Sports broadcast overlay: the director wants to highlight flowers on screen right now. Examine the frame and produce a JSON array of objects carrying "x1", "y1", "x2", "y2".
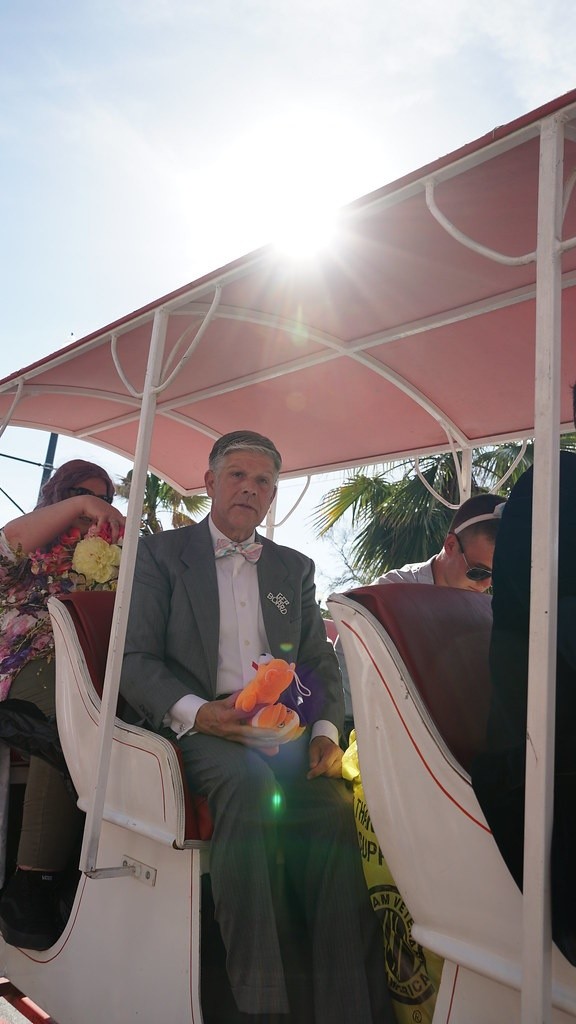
[{"x1": 3, "y1": 519, "x2": 129, "y2": 668}]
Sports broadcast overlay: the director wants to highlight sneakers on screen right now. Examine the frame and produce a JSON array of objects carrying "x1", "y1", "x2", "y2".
[{"x1": 0, "y1": 866, "x2": 64, "y2": 949}]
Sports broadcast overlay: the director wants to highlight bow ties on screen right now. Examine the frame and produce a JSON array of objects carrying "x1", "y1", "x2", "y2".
[{"x1": 214, "y1": 537, "x2": 265, "y2": 565}]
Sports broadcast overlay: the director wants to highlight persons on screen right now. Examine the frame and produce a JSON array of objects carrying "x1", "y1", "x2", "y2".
[
  {"x1": 118, "y1": 428, "x2": 397, "y2": 1024},
  {"x1": 1, "y1": 461, "x2": 131, "y2": 933},
  {"x1": 473, "y1": 381, "x2": 576, "y2": 963},
  {"x1": 332, "y1": 492, "x2": 506, "y2": 751}
]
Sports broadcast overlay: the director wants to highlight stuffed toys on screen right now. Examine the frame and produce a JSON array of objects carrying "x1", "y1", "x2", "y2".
[
  {"x1": 249, "y1": 703, "x2": 306, "y2": 745},
  {"x1": 235, "y1": 652, "x2": 296, "y2": 711}
]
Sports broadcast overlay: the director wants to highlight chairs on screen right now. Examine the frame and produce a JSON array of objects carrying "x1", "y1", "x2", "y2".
[
  {"x1": 47, "y1": 588, "x2": 224, "y2": 849},
  {"x1": 328, "y1": 575, "x2": 572, "y2": 1009}
]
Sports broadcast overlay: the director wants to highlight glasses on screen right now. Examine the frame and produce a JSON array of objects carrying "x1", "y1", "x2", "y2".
[
  {"x1": 447, "y1": 530, "x2": 495, "y2": 583},
  {"x1": 67, "y1": 486, "x2": 112, "y2": 506}
]
